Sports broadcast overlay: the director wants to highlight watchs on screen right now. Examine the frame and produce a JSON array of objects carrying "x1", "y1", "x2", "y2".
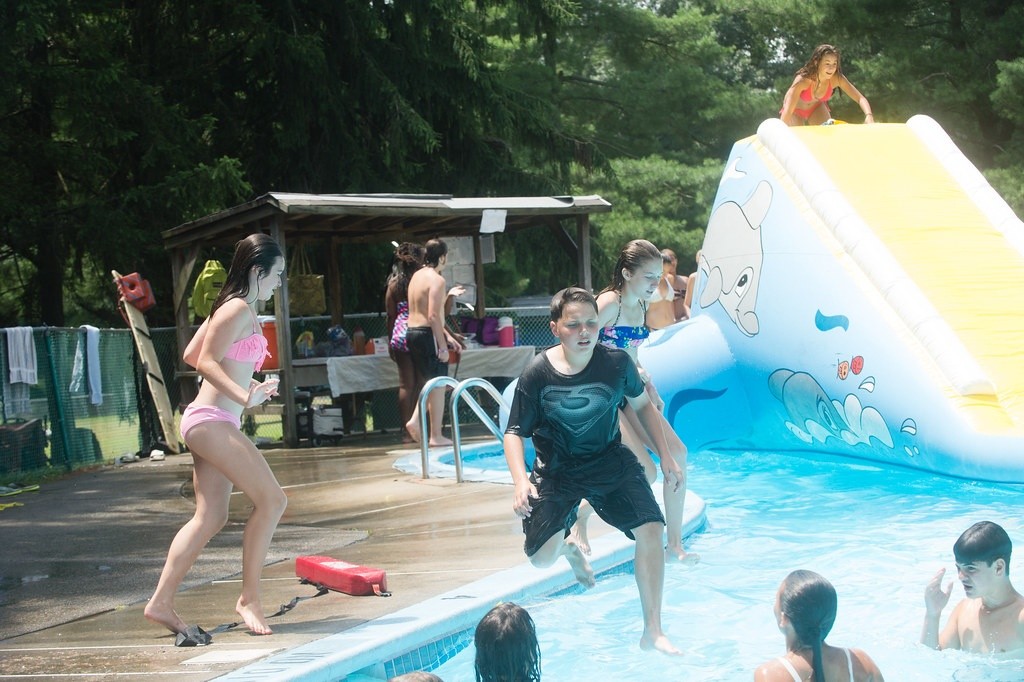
[{"x1": 438, "y1": 348, "x2": 449, "y2": 354}]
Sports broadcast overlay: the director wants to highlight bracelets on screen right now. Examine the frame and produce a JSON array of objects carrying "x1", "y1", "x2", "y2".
[{"x1": 864, "y1": 112, "x2": 873, "y2": 116}]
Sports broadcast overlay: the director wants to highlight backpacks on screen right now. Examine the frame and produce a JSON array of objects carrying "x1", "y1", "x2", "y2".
[{"x1": 191, "y1": 259, "x2": 229, "y2": 322}]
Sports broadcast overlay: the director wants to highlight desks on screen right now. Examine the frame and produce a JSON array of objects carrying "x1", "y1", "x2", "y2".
[{"x1": 287, "y1": 344, "x2": 537, "y2": 436}]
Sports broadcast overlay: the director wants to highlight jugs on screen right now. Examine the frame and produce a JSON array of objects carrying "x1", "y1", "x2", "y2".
[{"x1": 352, "y1": 326, "x2": 366, "y2": 355}]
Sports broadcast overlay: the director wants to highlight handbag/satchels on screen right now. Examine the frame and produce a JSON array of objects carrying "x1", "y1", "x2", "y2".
[
  {"x1": 459, "y1": 313, "x2": 504, "y2": 347},
  {"x1": 284, "y1": 246, "x2": 329, "y2": 315}
]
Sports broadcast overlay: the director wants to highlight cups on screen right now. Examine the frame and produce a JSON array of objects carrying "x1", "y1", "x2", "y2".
[{"x1": 296, "y1": 341, "x2": 308, "y2": 358}]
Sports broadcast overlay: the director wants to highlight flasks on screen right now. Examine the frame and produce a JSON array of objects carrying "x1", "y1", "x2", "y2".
[{"x1": 513, "y1": 321, "x2": 520, "y2": 346}]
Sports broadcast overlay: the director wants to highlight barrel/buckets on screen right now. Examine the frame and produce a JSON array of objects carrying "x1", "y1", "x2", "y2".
[{"x1": 496, "y1": 316, "x2": 514, "y2": 347}]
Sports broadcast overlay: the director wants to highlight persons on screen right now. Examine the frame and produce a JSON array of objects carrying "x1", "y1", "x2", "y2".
[
  {"x1": 499, "y1": 279, "x2": 687, "y2": 659},
  {"x1": 473, "y1": 600, "x2": 544, "y2": 682},
  {"x1": 141, "y1": 233, "x2": 290, "y2": 637},
  {"x1": 388, "y1": 669, "x2": 446, "y2": 682},
  {"x1": 778, "y1": 44, "x2": 875, "y2": 127},
  {"x1": 659, "y1": 249, "x2": 691, "y2": 323},
  {"x1": 642, "y1": 253, "x2": 677, "y2": 333},
  {"x1": 919, "y1": 520, "x2": 1024, "y2": 656},
  {"x1": 383, "y1": 255, "x2": 468, "y2": 443},
  {"x1": 567, "y1": 236, "x2": 704, "y2": 565},
  {"x1": 405, "y1": 238, "x2": 463, "y2": 447},
  {"x1": 685, "y1": 249, "x2": 702, "y2": 319},
  {"x1": 385, "y1": 241, "x2": 468, "y2": 442},
  {"x1": 753, "y1": 569, "x2": 886, "y2": 682}
]
